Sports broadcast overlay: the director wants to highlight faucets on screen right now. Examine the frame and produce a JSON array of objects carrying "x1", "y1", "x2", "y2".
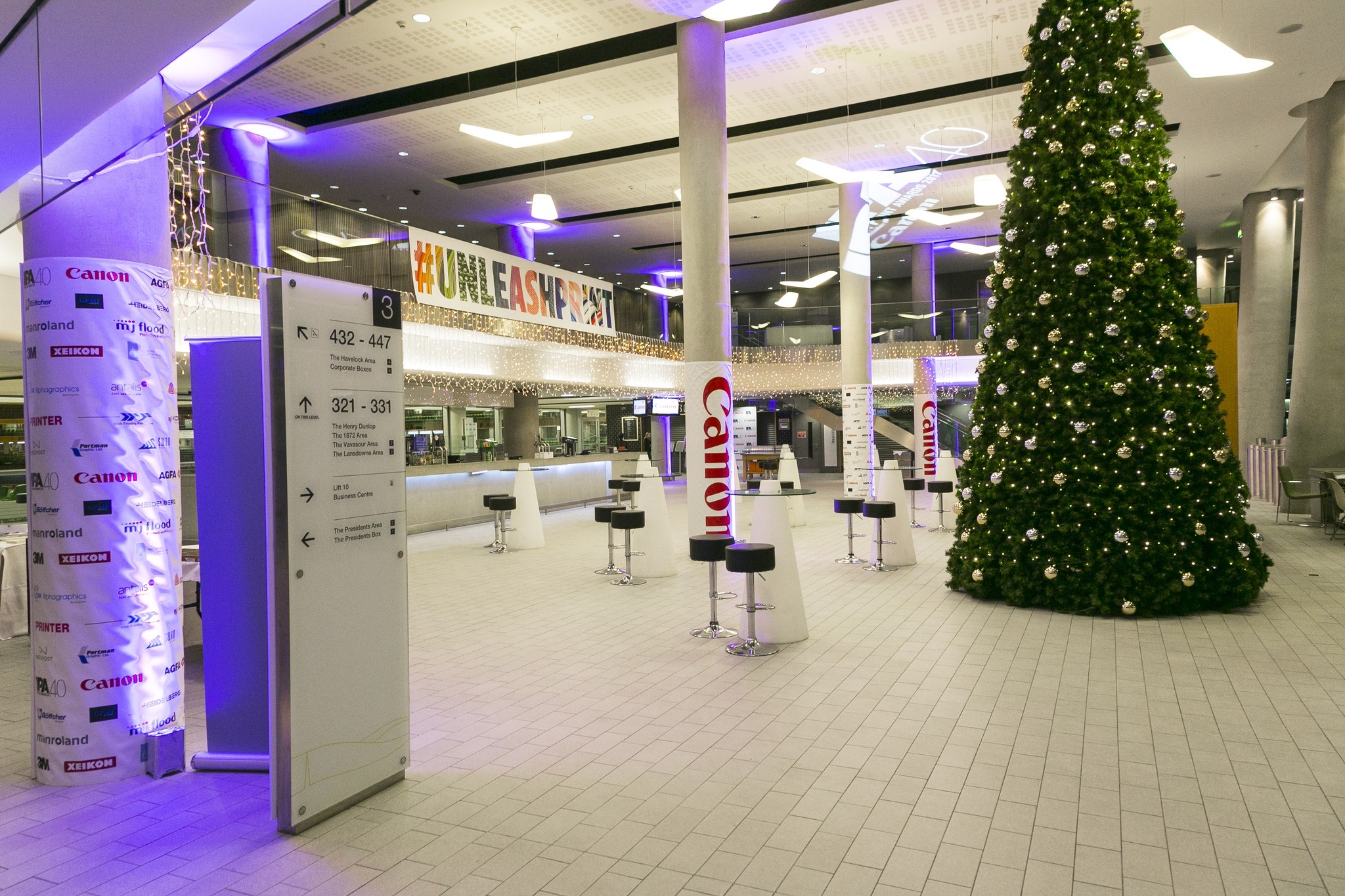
[{"x1": 554, "y1": 448, "x2": 565, "y2": 453}]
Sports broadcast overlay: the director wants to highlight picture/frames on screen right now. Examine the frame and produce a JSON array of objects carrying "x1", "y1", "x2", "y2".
[{"x1": 780, "y1": 422, "x2": 789, "y2": 429}]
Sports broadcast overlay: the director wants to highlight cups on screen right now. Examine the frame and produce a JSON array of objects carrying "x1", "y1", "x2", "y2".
[{"x1": 504, "y1": 453, "x2": 509, "y2": 460}]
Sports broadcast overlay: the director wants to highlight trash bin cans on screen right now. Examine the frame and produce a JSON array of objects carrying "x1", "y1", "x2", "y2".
[{"x1": 894, "y1": 451, "x2": 912, "y2": 479}]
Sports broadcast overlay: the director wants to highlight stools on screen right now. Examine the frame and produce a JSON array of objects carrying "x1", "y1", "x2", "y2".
[
  {"x1": 759, "y1": 461, "x2": 777, "y2": 479},
  {"x1": 780, "y1": 481, "x2": 795, "y2": 529},
  {"x1": 903, "y1": 479, "x2": 955, "y2": 532},
  {"x1": 689, "y1": 535, "x2": 780, "y2": 656},
  {"x1": 595, "y1": 480, "x2": 647, "y2": 586},
  {"x1": 483, "y1": 494, "x2": 518, "y2": 554},
  {"x1": 834, "y1": 498, "x2": 898, "y2": 572}
]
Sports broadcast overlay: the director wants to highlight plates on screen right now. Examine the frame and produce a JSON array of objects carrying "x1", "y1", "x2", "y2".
[{"x1": 618, "y1": 450, "x2": 628, "y2": 452}]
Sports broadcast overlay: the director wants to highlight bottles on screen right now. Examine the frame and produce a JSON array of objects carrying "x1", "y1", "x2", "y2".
[
  {"x1": 409, "y1": 450, "x2": 435, "y2": 466},
  {"x1": 16, "y1": 424, "x2": 24, "y2": 435}
]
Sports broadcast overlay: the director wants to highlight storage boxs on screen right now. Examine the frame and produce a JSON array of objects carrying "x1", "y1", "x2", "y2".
[{"x1": 749, "y1": 461, "x2": 765, "y2": 472}]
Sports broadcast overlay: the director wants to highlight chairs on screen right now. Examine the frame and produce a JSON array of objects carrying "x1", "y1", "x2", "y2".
[{"x1": 1276, "y1": 465, "x2": 1345, "y2": 545}]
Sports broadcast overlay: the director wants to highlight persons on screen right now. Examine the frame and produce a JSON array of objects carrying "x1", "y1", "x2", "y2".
[
  {"x1": 682, "y1": 435, "x2": 686, "y2": 468},
  {"x1": 643, "y1": 432, "x2": 652, "y2": 461}
]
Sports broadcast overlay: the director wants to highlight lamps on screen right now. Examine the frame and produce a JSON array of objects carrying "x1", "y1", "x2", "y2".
[{"x1": 531, "y1": 118, "x2": 558, "y2": 220}]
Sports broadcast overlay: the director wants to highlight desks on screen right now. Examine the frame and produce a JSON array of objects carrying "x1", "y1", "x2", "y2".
[
  {"x1": 858, "y1": 466, "x2": 923, "y2": 566},
  {"x1": 620, "y1": 473, "x2": 683, "y2": 578},
  {"x1": 500, "y1": 468, "x2": 549, "y2": 550},
  {"x1": 1309, "y1": 468, "x2": 1345, "y2": 521},
  {"x1": 741, "y1": 453, "x2": 780, "y2": 482},
  {"x1": 626, "y1": 458, "x2": 664, "y2": 507},
  {"x1": 770, "y1": 457, "x2": 811, "y2": 526},
  {"x1": 724, "y1": 489, "x2": 817, "y2": 643}
]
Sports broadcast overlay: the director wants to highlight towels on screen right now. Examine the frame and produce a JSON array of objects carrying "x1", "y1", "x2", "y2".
[{"x1": 893, "y1": 450, "x2": 908, "y2": 456}]
